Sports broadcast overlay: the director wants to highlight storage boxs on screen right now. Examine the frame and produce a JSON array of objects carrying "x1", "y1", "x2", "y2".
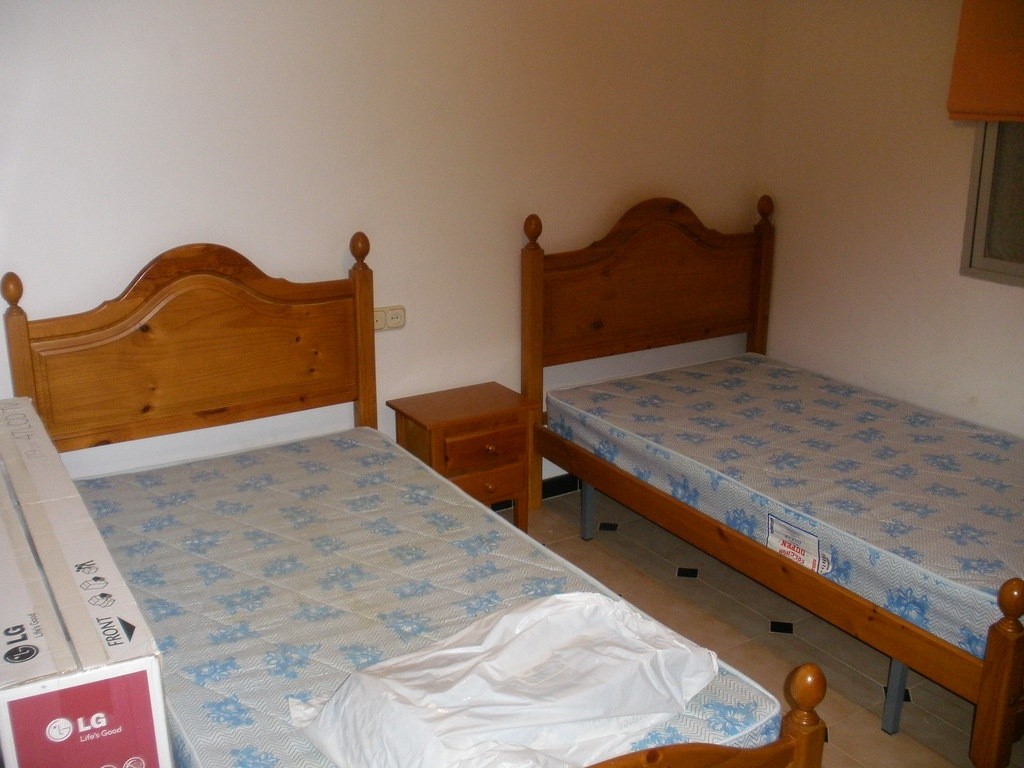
[{"x1": 0, "y1": 396, "x2": 173, "y2": 767}]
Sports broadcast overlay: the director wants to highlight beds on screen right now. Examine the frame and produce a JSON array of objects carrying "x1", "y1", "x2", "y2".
[
  {"x1": 1, "y1": 227, "x2": 825, "y2": 767},
  {"x1": 517, "y1": 189, "x2": 1023, "y2": 768}
]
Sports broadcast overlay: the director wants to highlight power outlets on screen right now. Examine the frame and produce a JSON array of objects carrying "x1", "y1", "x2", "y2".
[{"x1": 374, "y1": 304, "x2": 407, "y2": 333}]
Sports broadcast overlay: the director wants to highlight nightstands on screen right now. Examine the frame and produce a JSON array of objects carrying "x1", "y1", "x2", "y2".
[{"x1": 386, "y1": 382, "x2": 540, "y2": 534}]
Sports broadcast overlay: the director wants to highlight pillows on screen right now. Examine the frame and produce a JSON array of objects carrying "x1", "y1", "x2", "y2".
[{"x1": 288, "y1": 590, "x2": 722, "y2": 768}]
